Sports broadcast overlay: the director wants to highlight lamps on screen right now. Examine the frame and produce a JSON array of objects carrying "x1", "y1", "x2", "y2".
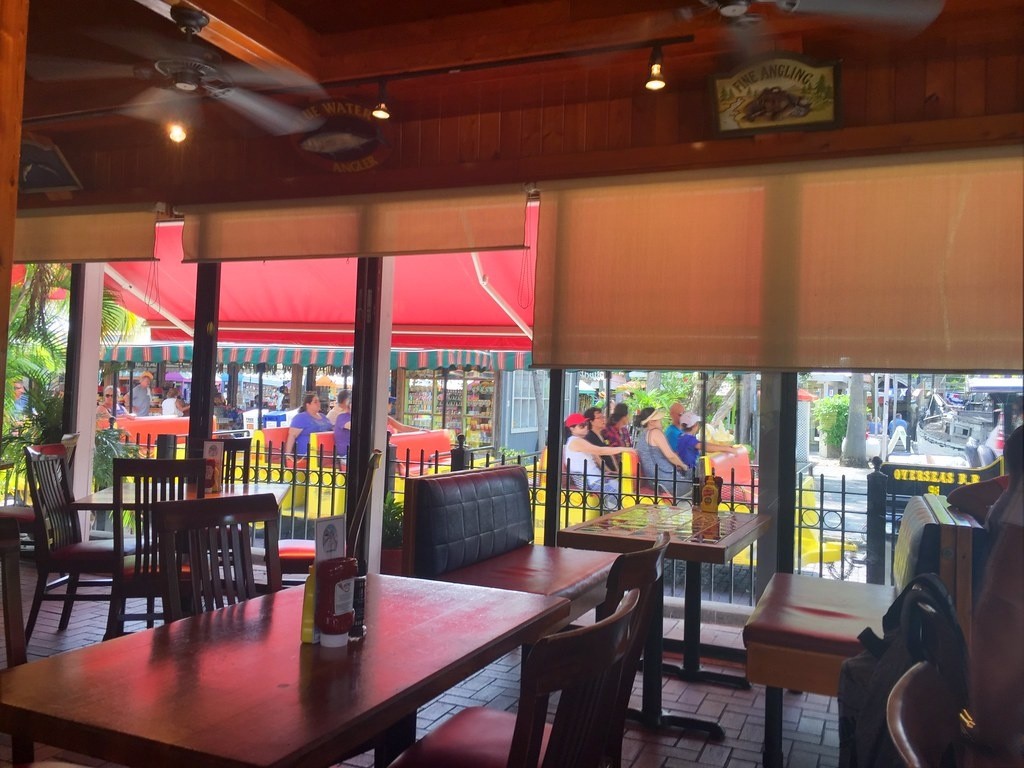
[
  {"x1": 644, "y1": 42, "x2": 665, "y2": 92},
  {"x1": 372, "y1": 79, "x2": 390, "y2": 120}
]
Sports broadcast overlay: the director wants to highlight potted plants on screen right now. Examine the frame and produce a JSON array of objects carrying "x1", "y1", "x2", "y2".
[{"x1": 813, "y1": 392, "x2": 871, "y2": 460}]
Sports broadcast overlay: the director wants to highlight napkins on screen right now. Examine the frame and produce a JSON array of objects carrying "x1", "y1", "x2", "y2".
[{"x1": 964, "y1": 442, "x2": 996, "y2": 468}]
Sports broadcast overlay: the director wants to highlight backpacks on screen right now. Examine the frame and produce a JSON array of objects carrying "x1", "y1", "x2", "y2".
[{"x1": 839, "y1": 570, "x2": 968, "y2": 768}]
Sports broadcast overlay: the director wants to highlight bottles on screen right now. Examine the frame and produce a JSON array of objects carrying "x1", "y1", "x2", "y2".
[
  {"x1": 300, "y1": 556, "x2": 369, "y2": 648},
  {"x1": 691, "y1": 477, "x2": 723, "y2": 513},
  {"x1": 205, "y1": 459, "x2": 219, "y2": 495}
]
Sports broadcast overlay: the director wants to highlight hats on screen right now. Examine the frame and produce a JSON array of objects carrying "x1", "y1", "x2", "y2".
[
  {"x1": 565, "y1": 415, "x2": 590, "y2": 428},
  {"x1": 639, "y1": 412, "x2": 666, "y2": 425},
  {"x1": 679, "y1": 413, "x2": 702, "y2": 428}
]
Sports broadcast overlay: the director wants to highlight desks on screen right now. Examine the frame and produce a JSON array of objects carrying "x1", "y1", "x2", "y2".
[
  {"x1": 557, "y1": 503, "x2": 772, "y2": 741},
  {"x1": 0, "y1": 573, "x2": 568, "y2": 768},
  {"x1": 70, "y1": 484, "x2": 290, "y2": 509}
]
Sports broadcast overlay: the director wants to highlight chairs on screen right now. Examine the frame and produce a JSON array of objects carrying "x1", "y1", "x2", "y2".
[
  {"x1": 0, "y1": 431, "x2": 672, "y2": 768},
  {"x1": 886, "y1": 657, "x2": 971, "y2": 768}
]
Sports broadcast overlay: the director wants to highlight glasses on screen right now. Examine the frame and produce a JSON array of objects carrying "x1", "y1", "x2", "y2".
[
  {"x1": 592, "y1": 416, "x2": 606, "y2": 421},
  {"x1": 574, "y1": 422, "x2": 589, "y2": 429},
  {"x1": 105, "y1": 394, "x2": 113, "y2": 398}
]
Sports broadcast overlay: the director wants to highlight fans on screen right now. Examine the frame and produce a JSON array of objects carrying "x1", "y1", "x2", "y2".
[
  {"x1": 697, "y1": 0, "x2": 945, "y2": 28},
  {"x1": 25, "y1": 5, "x2": 328, "y2": 137}
]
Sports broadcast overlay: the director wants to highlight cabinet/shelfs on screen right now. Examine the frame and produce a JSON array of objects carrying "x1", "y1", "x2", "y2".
[{"x1": 404, "y1": 371, "x2": 494, "y2": 447}]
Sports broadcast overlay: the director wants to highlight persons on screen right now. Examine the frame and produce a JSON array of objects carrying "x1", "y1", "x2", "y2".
[
  {"x1": 582, "y1": 407, "x2": 620, "y2": 479},
  {"x1": 604, "y1": 403, "x2": 632, "y2": 468},
  {"x1": 162, "y1": 388, "x2": 190, "y2": 416},
  {"x1": 985, "y1": 412, "x2": 1004, "y2": 457},
  {"x1": 334, "y1": 398, "x2": 352, "y2": 464},
  {"x1": 253, "y1": 394, "x2": 259, "y2": 407},
  {"x1": 947, "y1": 424, "x2": 1024, "y2": 767},
  {"x1": 866, "y1": 414, "x2": 882, "y2": 434},
  {"x1": 889, "y1": 413, "x2": 908, "y2": 438},
  {"x1": 665, "y1": 402, "x2": 686, "y2": 452},
  {"x1": 12, "y1": 379, "x2": 28, "y2": 418},
  {"x1": 285, "y1": 393, "x2": 334, "y2": 460},
  {"x1": 564, "y1": 414, "x2": 637, "y2": 513},
  {"x1": 327, "y1": 390, "x2": 351, "y2": 425},
  {"x1": 123, "y1": 376, "x2": 150, "y2": 416},
  {"x1": 276, "y1": 386, "x2": 290, "y2": 409},
  {"x1": 677, "y1": 412, "x2": 737, "y2": 468},
  {"x1": 387, "y1": 396, "x2": 430, "y2": 434},
  {"x1": 635, "y1": 406, "x2": 695, "y2": 497},
  {"x1": 96, "y1": 385, "x2": 128, "y2": 417}
]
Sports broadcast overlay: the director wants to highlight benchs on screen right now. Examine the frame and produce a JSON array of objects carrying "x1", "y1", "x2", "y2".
[
  {"x1": 400, "y1": 445, "x2": 990, "y2": 768},
  {"x1": 96, "y1": 415, "x2": 454, "y2": 473}
]
